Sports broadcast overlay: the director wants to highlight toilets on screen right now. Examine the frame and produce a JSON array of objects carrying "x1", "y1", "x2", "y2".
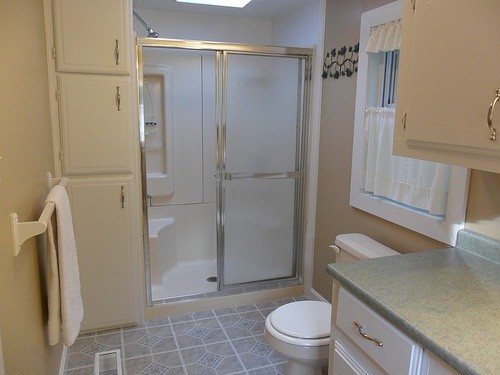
[{"x1": 263, "y1": 233, "x2": 404, "y2": 375}]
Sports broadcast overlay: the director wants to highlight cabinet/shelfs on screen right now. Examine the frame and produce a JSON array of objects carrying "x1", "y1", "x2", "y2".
[
  {"x1": 326, "y1": 248, "x2": 500, "y2": 375},
  {"x1": 61, "y1": 178, "x2": 147, "y2": 328},
  {"x1": 392, "y1": 0, "x2": 500, "y2": 173},
  {"x1": 53, "y1": 0, "x2": 130, "y2": 75},
  {"x1": 55, "y1": 73, "x2": 137, "y2": 174}
]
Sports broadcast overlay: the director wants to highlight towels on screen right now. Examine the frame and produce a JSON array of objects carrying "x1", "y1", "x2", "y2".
[{"x1": 40, "y1": 184, "x2": 86, "y2": 349}]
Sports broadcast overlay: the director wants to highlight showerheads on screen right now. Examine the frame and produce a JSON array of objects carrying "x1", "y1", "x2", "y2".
[{"x1": 133, "y1": 6, "x2": 161, "y2": 38}]
